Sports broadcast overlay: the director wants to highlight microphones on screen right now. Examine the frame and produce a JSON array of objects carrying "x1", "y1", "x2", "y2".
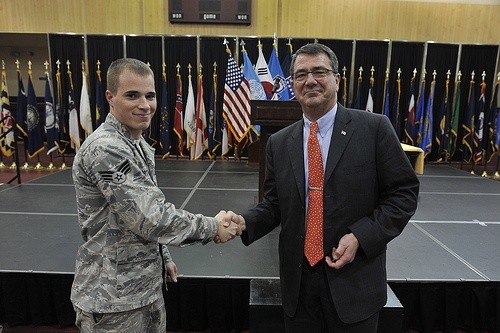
[{"x1": 272, "y1": 76, "x2": 281, "y2": 93}]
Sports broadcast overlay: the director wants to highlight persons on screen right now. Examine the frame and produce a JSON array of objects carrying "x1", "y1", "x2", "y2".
[
  {"x1": 69, "y1": 58, "x2": 243, "y2": 333},
  {"x1": 224, "y1": 44, "x2": 420, "y2": 333}
]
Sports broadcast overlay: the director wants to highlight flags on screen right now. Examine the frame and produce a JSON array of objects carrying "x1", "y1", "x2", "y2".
[
  {"x1": 222, "y1": 55, "x2": 254, "y2": 142},
  {"x1": 254, "y1": 48, "x2": 278, "y2": 100},
  {"x1": 267, "y1": 45, "x2": 295, "y2": 101},
  {"x1": 142, "y1": 72, "x2": 221, "y2": 161},
  {"x1": 240, "y1": 52, "x2": 268, "y2": 136},
  {"x1": 282, "y1": 47, "x2": 298, "y2": 100},
  {"x1": 338, "y1": 75, "x2": 500, "y2": 179},
  {"x1": 0, "y1": 69, "x2": 106, "y2": 157}
]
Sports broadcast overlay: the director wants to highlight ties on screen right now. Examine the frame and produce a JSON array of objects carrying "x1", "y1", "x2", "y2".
[{"x1": 303, "y1": 123, "x2": 324, "y2": 267}]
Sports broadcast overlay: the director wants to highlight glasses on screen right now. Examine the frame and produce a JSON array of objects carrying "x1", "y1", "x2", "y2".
[{"x1": 291, "y1": 68, "x2": 339, "y2": 83}]
